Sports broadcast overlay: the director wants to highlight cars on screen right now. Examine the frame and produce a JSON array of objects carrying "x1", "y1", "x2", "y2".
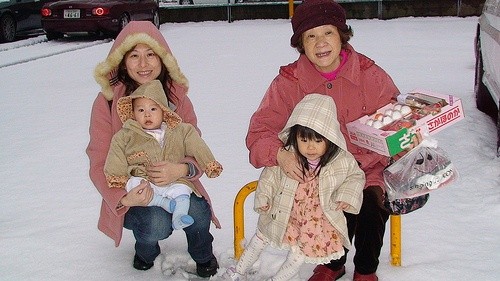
[
  {"x1": 0, "y1": 0, "x2": 51, "y2": 43},
  {"x1": 40, "y1": 0, "x2": 161, "y2": 41},
  {"x1": 472, "y1": 0, "x2": 500, "y2": 156}
]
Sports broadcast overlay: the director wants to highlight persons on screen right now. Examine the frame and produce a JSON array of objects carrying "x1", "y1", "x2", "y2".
[
  {"x1": 85, "y1": 19, "x2": 222, "y2": 277},
  {"x1": 104, "y1": 80, "x2": 224, "y2": 229},
  {"x1": 222, "y1": 92, "x2": 365, "y2": 281},
  {"x1": 244, "y1": 0, "x2": 423, "y2": 281}
]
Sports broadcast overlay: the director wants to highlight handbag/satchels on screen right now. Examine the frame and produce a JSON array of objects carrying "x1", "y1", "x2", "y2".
[
  {"x1": 383, "y1": 135, "x2": 458, "y2": 201},
  {"x1": 382, "y1": 161, "x2": 429, "y2": 215}
]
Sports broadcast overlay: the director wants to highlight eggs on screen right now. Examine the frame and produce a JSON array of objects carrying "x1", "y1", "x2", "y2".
[{"x1": 365, "y1": 104, "x2": 412, "y2": 129}]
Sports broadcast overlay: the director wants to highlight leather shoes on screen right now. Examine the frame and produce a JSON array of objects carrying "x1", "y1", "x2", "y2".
[
  {"x1": 196, "y1": 255, "x2": 219, "y2": 277},
  {"x1": 134, "y1": 245, "x2": 160, "y2": 270}
]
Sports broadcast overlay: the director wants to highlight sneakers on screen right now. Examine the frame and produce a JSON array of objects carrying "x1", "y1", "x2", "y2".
[{"x1": 222, "y1": 267, "x2": 247, "y2": 281}]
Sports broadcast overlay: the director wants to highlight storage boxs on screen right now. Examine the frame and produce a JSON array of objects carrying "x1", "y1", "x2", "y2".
[{"x1": 345, "y1": 87, "x2": 465, "y2": 156}]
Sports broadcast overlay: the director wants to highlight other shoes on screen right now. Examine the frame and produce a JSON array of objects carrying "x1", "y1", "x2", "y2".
[
  {"x1": 308, "y1": 264, "x2": 345, "y2": 281},
  {"x1": 353, "y1": 270, "x2": 378, "y2": 281}
]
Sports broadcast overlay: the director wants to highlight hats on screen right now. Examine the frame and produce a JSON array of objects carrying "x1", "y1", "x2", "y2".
[{"x1": 291, "y1": 0, "x2": 348, "y2": 47}]
